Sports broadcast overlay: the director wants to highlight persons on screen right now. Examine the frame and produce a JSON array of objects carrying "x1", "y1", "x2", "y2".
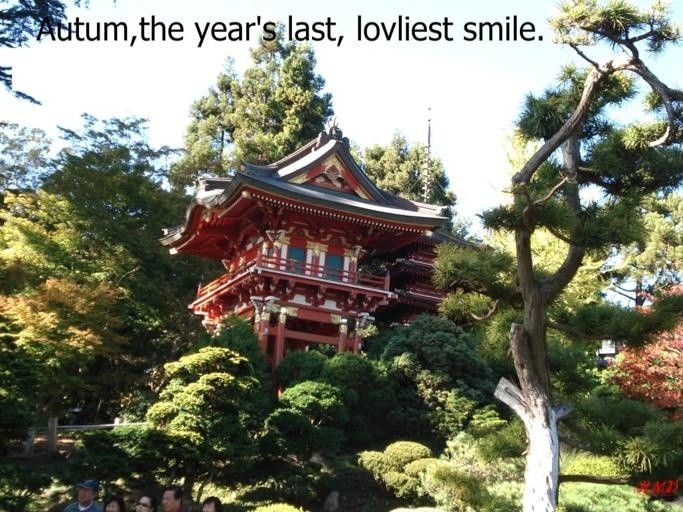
[
  {"x1": 198, "y1": 495, "x2": 222, "y2": 511},
  {"x1": 133, "y1": 493, "x2": 159, "y2": 511},
  {"x1": 100, "y1": 493, "x2": 128, "y2": 511},
  {"x1": 62, "y1": 478, "x2": 103, "y2": 511},
  {"x1": 161, "y1": 484, "x2": 186, "y2": 510}
]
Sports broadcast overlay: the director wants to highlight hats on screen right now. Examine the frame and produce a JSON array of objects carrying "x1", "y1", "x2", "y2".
[{"x1": 77, "y1": 480, "x2": 97, "y2": 490}]
